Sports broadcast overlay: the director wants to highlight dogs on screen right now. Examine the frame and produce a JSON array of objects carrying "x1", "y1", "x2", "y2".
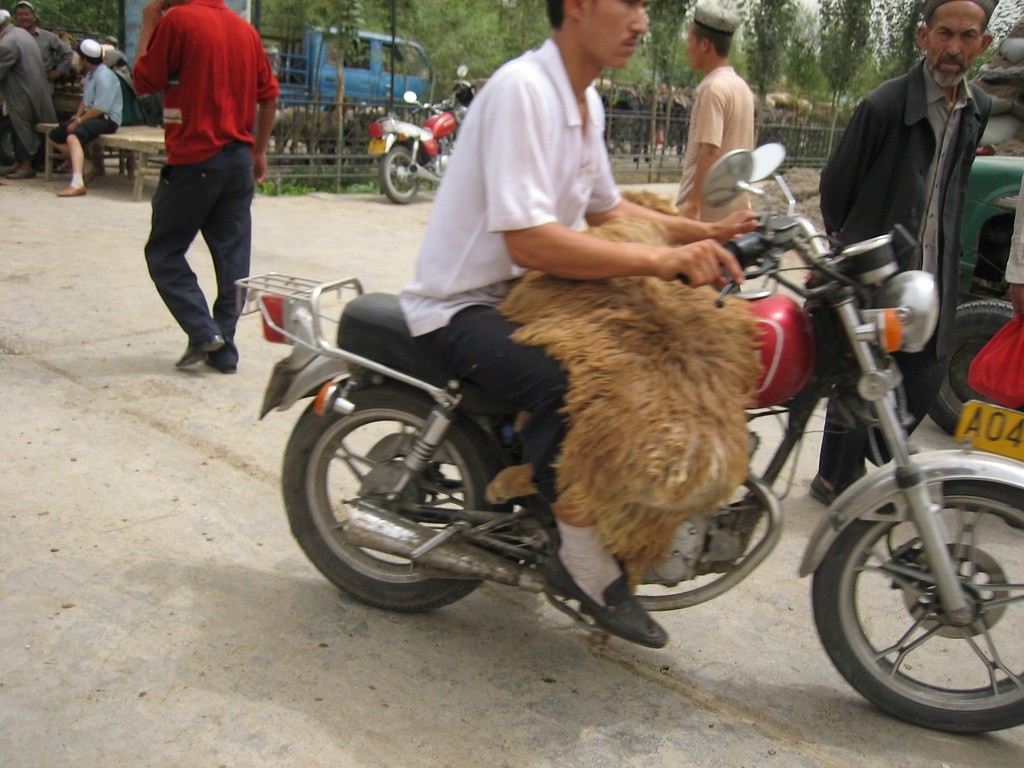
[{"x1": 485, "y1": 189, "x2": 767, "y2": 586}]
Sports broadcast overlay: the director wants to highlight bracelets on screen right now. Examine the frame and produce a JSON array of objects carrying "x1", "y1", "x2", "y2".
[{"x1": 78, "y1": 117, "x2": 82, "y2": 124}]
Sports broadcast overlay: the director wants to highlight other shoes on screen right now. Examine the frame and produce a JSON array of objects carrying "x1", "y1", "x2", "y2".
[
  {"x1": 0, "y1": 169, "x2": 17, "y2": 176},
  {"x1": 57, "y1": 185, "x2": 85, "y2": 197},
  {"x1": 7, "y1": 170, "x2": 35, "y2": 178},
  {"x1": 54, "y1": 161, "x2": 73, "y2": 173},
  {"x1": 807, "y1": 474, "x2": 896, "y2": 515}
]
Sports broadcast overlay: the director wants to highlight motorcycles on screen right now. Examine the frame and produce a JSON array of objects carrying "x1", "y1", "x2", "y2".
[
  {"x1": 236, "y1": 141, "x2": 1024, "y2": 734},
  {"x1": 367, "y1": 64, "x2": 475, "y2": 204}
]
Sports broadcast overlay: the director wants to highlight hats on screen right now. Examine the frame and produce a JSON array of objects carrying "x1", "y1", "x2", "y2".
[
  {"x1": 925, "y1": 0, "x2": 999, "y2": 24},
  {"x1": 0, "y1": 8, "x2": 12, "y2": 27},
  {"x1": 104, "y1": 36, "x2": 121, "y2": 47},
  {"x1": 14, "y1": 1, "x2": 41, "y2": 27},
  {"x1": 76, "y1": 39, "x2": 102, "y2": 64},
  {"x1": 693, "y1": 4, "x2": 742, "y2": 35}
]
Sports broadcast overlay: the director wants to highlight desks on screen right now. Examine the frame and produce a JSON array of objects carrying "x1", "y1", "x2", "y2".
[{"x1": 36, "y1": 121, "x2": 167, "y2": 201}]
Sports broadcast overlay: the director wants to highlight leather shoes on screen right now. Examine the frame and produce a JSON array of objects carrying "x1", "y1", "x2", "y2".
[
  {"x1": 545, "y1": 550, "x2": 668, "y2": 651},
  {"x1": 176, "y1": 330, "x2": 225, "y2": 370},
  {"x1": 205, "y1": 361, "x2": 236, "y2": 374}
]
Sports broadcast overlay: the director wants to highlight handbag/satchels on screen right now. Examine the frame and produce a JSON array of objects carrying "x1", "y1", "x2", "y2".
[{"x1": 968, "y1": 314, "x2": 1024, "y2": 408}]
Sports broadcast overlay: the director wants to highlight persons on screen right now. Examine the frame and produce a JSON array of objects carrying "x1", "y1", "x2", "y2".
[
  {"x1": 397, "y1": 0, "x2": 759, "y2": 649},
  {"x1": 1003, "y1": 169, "x2": 1024, "y2": 324},
  {"x1": 130, "y1": 0, "x2": 279, "y2": 375},
  {"x1": 677, "y1": 0, "x2": 755, "y2": 225},
  {"x1": 0, "y1": 0, "x2": 139, "y2": 185},
  {"x1": 45, "y1": 38, "x2": 124, "y2": 197},
  {"x1": 808, "y1": 0, "x2": 999, "y2": 525}
]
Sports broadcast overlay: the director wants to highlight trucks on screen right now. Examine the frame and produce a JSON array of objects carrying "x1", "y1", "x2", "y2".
[
  {"x1": 262, "y1": 22, "x2": 436, "y2": 113},
  {"x1": 925, "y1": 64, "x2": 1024, "y2": 435}
]
